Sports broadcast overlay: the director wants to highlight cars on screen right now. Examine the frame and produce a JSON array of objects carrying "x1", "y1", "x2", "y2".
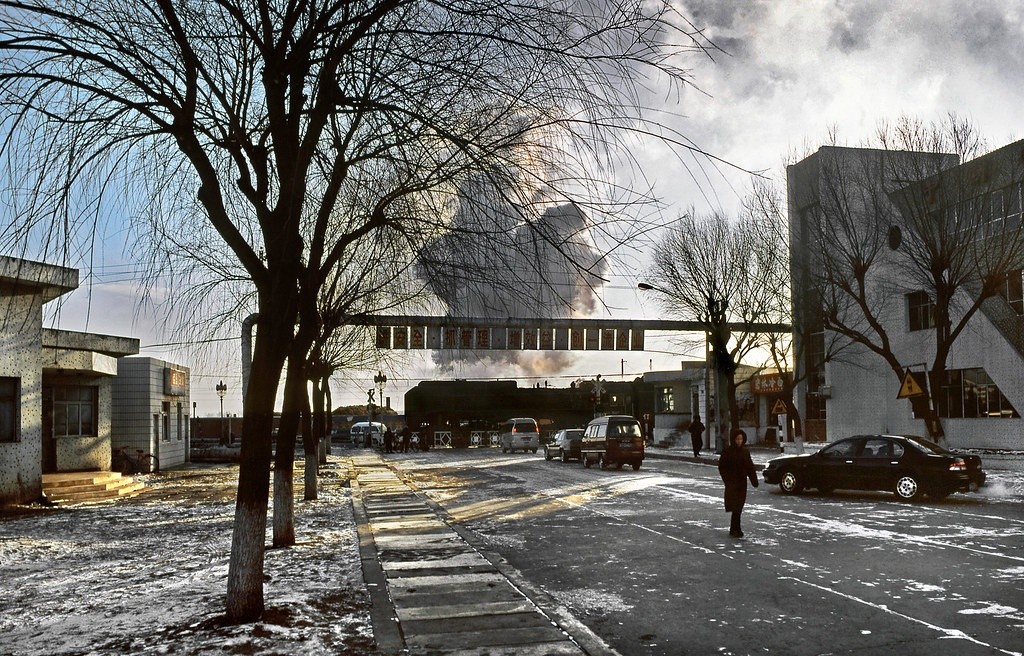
[
  {"x1": 544, "y1": 429, "x2": 584, "y2": 463},
  {"x1": 761, "y1": 434, "x2": 989, "y2": 503}
]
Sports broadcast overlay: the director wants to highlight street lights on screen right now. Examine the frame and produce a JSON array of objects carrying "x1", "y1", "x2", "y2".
[
  {"x1": 374, "y1": 370, "x2": 388, "y2": 451},
  {"x1": 215, "y1": 380, "x2": 228, "y2": 448},
  {"x1": 638, "y1": 283, "x2": 714, "y2": 452}
]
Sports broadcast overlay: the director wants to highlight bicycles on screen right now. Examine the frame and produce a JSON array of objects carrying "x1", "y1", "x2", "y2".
[
  {"x1": 111, "y1": 446, "x2": 161, "y2": 473},
  {"x1": 390, "y1": 436, "x2": 419, "y2": 453}
]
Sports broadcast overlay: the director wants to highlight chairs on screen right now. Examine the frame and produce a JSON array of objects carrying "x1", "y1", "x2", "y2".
[{"x1": 862, "y1": 445, "x2": 889, "y2": 457}]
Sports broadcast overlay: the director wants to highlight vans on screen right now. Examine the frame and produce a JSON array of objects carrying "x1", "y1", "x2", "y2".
[
  {"x1": 580, "y1": 414, "x2": 646, "y2": 469},
  {"x1": 349, "y1": 422, "x2": 388, "y2": 446},
  {"x1": 499, "y1": 417, "x2": 540, "y2": 453}
]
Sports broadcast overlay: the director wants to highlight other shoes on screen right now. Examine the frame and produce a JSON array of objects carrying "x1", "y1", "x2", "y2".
[{"x1": 730, "y1": 530, "x2": 743, "y2": 536}]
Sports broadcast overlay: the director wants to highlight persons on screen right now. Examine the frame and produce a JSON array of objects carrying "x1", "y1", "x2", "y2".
[
  {"x1": 399, "y1": 425, "x2": 410, "y2": 453},
  {"x1": 718, "y1": 428, "x2": 759, "y2": 538},
  {"x1": 383, "y1": 426, "x2": 395, "y2": 453},
  {"x1": 689, "y1": 415, "x2": 705, "y2": 457}
]
